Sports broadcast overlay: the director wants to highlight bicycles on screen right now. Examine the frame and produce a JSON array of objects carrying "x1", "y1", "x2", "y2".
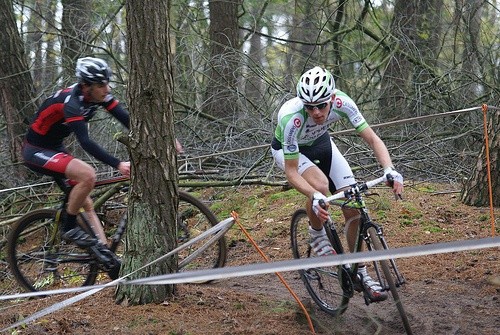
[
  {"x1": 289, "y1": 172, "x2": 415, "y2": 335},
  {"x1": 6, "y1": 162, "x2": 228, "y2": 299}
]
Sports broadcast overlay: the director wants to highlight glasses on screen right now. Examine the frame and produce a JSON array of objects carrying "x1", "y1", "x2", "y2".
[{"x1": 304, "y1": 101, "x2": 330, "y2": 111}]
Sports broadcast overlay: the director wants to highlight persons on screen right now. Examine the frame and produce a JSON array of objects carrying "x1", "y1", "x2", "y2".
[
  {"x1": 271, "y1": 66, "x2": 404, "y2": 295},
  {"x1": 22, "y1": 56, "x2": 182, "y2": 248}
]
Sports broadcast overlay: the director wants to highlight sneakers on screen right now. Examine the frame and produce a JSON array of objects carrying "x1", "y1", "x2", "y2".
[
  {"x1": 309, "y1": 234, "x2": 335, "y2": 258},
  {"x1": 61, "y1": 228, "x2": 97, "y2": 246},
  {"x1": 362, "y1": 279, "x2": 388, "y2": 302},
  {"x1": 109, "y1": 258, "x2": 124, "y2": 281}
]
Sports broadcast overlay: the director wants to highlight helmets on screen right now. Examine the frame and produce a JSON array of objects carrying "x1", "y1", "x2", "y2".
[
  {"x1": 296, "y1": 67, "x2": 335, "y2": 105},
  {"x1": 76, "y1": 57, "x2": 110, "y2": 86}
]
